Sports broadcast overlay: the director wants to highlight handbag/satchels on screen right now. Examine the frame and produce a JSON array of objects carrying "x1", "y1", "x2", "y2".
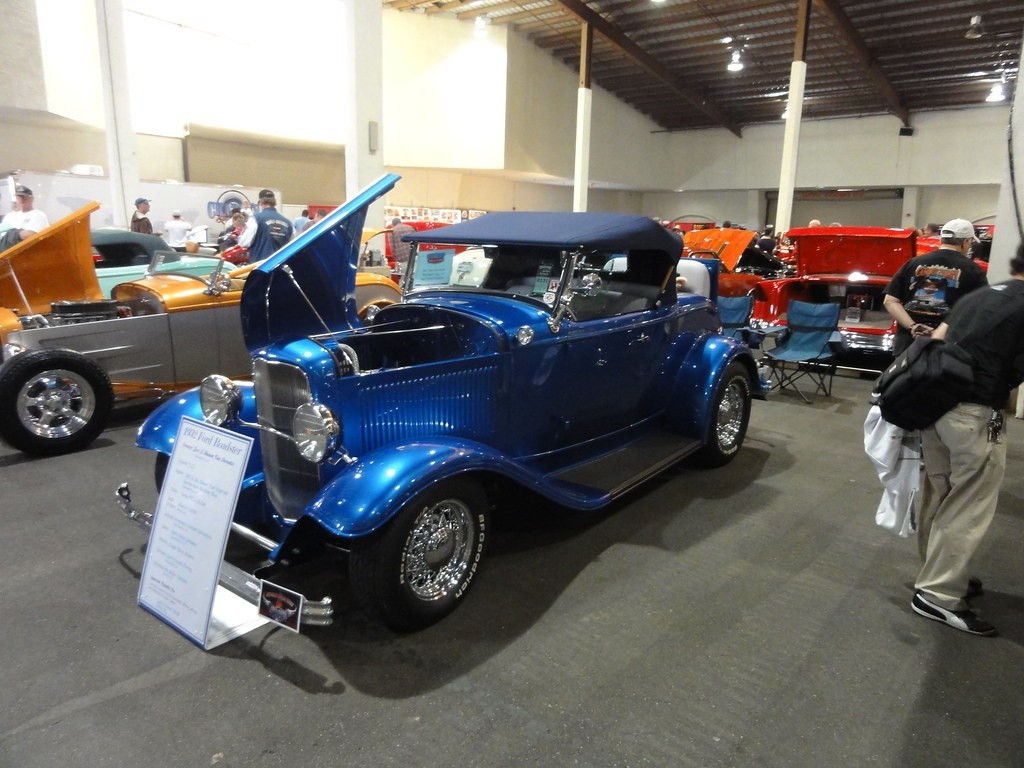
[{"x1": 872, "y1": 334, "x2": 975, "y2": 431}]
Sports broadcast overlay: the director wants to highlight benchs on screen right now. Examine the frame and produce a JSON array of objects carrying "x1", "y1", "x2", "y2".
[{"x1": 518, "y1": 276, "x2": 662, "y2": 303}]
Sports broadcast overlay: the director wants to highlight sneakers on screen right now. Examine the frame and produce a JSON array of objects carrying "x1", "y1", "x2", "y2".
[
  {"x1": 965, "y1": 576, "x2": 983, "y2": 597},
  {"x1": 911, "y1": 592, "x2": 993, "y2": 635}
]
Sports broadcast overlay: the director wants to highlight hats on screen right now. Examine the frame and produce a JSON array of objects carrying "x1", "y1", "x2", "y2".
[
  {"x1": 134, "y1": 198, "x2": 152, "y2": 205},
  {"x1": 941, "y1": 219, "x2": 981, "y2": 243},
  {"x1": 14, "y1": 186, "x2": 32, "y2": 196},
  {"x1": 259, "y1": 190, "x2": 274, "y2": 198},
  {"x1": 673, "y1": 224, "x2": 680, "y2": 230},
  {"x1": 172, "y1": 211, "x2": 179, "y2": 216}
]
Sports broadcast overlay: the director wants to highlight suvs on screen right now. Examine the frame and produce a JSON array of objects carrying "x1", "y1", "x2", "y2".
[{"x1": 111, "y1": 171, "x2": 792, "y2": 626}]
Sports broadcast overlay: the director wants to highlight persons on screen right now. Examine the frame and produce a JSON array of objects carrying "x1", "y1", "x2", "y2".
[
  {"x1": 884, "y1": 219, "x2": 989, "y2": 360},
  {"x1": 722, "y1": 220, "x2": 746, "y2": 230},
  {"x1": 164, "y1": 212, "x2": 192, "y2": 252},
  {"x1": 131, "y1": 198, "x2": 162, "y2": 237},
  {"x1": 756, "y1": 225, "x2": 782, "y2": 254},
  {"x1": 0, "y1": 186, "x2": 50, "y2": 241},
  {"x1": 673, "y1": 225, "x2": 684, "y2": 238},
  {"x1": 388, "y1": 218, "x2": 418, "y2": 287},
  {"x1": 830, "y1": 222, "x2": 842, "y2": 227},
  {"x1": 924, "y1": 223, "x2": 941, "y2": 239},
  {"x1": 293, "y1": 210, "x2": 310, "y2": 232},
  {"x1": 223, "y1": 200, "x2": 262, "y2": 236},
  {"x1": 239, "y1": 189, "x2": 296, "y2": 263},
  {"x1": 809, "y1": 219, "x2": 820, "y2": 227},
  {"x1": 909, "y1": 244, "x2": 1024, "y2": 639},
  {"x1": 302, "y1": 209, "x2": 327, "y2": 232}
]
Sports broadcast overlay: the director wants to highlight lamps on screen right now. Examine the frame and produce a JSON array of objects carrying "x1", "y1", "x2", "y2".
[
  {"x1": 899, "y1": 127, "x2": 912, "y2": 136},
  {"x1": 369, "y1": 120, "x2": 378, "y2": 150}
]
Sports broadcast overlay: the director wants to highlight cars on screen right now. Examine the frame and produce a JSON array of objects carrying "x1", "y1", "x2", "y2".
[
  {"x1": 0, "y1": 197, "x2": 406, "y2": 458},
  {"x1": 657, "y1": 217, "x2": 995, "y2": 378},
  {"x1": 0, "y1": 226, "x2": 238, "y2": 299},
  {"x1": 304, "y1": 204, "x2": 470, "y2": 290}
]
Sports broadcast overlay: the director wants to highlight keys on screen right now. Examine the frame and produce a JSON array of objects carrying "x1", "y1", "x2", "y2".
[{"x1": 988, "y1": 420, "x2": 1002, "y2": 442}]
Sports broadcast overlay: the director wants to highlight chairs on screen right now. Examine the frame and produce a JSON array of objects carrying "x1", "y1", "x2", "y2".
[
  {"x1": 714, "y1": 295, "x2": 843, "y2": 404},
  {"x1": 563, "y1": 286, "x2": 651, "y2": 323},
  {"x1": 505, "y1": 284, "x2": 555, "y2": 308}
]
